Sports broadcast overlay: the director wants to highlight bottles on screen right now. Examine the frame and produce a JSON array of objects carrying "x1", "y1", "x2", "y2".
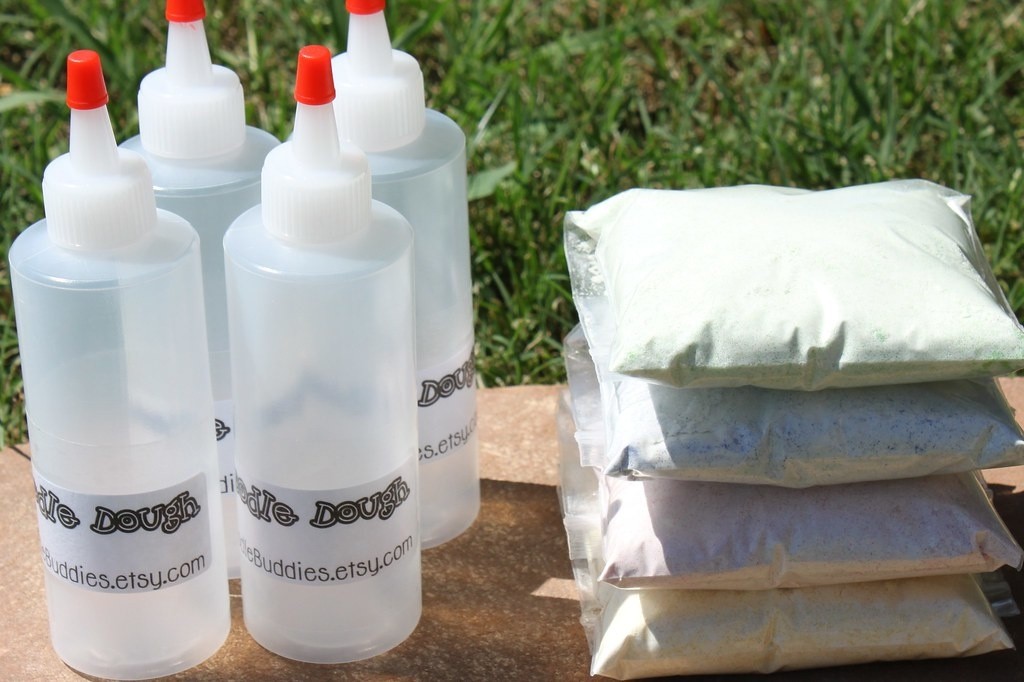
[
  {"x1": 328, "y1": 0, "x2": 479, "y2": 548},
  {"x1": 8, "y1": 50, "x2": 230, "y2": 681},
  {"x1": 222, "y1": 45, "x2": 423, "y2": 666},
  {"x1": 117, "y1": 0, "x2": 282, "y2": 581}
]
